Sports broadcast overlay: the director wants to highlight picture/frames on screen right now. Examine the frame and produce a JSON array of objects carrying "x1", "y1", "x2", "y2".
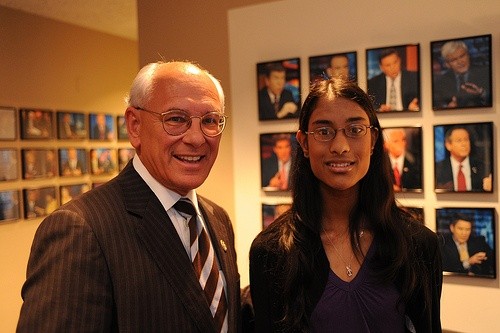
[
  {"x1": 0, "y1": 106, "x2": 136, "y2": 226},
  {"x1": 255, "y1": 34, "x2": 497, "y2": 279}
]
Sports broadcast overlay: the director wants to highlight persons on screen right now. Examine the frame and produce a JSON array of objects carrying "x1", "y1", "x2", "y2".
[
  {"x1": 20, "y1": 107, "x2": 136, "y2": 220},
  {"x1": 15, "y1": 60, "x2": 242, "y2": 333},
  {"x1": 260, "y1": 41, "x2": 495, "y2": 279},
  {"x1": 249, "y1": 77, "x2": 444, "y2": 333}
]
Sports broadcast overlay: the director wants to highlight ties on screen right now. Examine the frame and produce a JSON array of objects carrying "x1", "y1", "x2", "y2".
[
  {"x1": 389, "y1": 79, "x2": 396, "y2": 112},
  {"x1": 459, "y1": 75, "x2": 466, "y2": 98},
  {"x1": 457, "y1": 161, "x2": 468, "y2": 192},
  {"x1": 173, "y1": 198, "x2": 231, "y2": 333},
  {"x1": 459, "y1": 245, "x2": 468, "y2": 263},
  {"x1": 279, "y1": 163, "x2": 287, "y2": 190},
  {"x1": 393, "y1": 162, "x2": 401, "y2": 188},
  {"x1": 273, "y1": 95, "x2": 279, "y2": 111}
]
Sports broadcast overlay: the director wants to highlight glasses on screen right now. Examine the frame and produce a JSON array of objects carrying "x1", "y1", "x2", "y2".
[
  {"x1": 134, "y1": 107, "x2": 228, "y2": 137},
  {"x1": 302, "y1": 123, "x2": 374, "y2": 143}
]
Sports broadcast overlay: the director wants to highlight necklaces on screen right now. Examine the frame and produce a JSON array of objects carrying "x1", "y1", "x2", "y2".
[{"x1": 318, "y1": 216, "x2": 366, "y2": 277}]
